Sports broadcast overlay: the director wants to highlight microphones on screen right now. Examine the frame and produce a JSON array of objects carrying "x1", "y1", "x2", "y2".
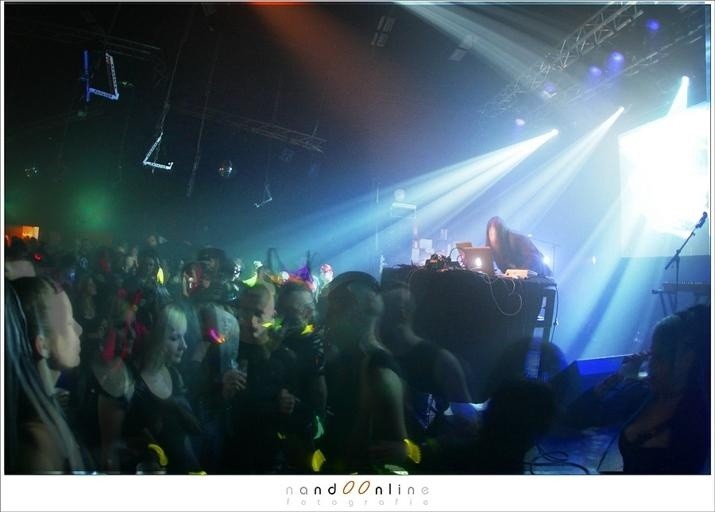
[{"x1": 697, "y1": 211, "x2": 709, "y2": 229}]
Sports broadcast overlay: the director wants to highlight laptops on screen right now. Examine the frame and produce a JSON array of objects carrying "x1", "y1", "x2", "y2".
[
  {"x1": 456, "y1": 242, "x2": 473, "y2": 260},
  {"x1": 464, "y1": 247, "x2": 495, "y2": 275}
]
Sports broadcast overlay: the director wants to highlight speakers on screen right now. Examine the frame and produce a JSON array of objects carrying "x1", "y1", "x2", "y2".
[{"x1": 547, "y1": 355, "x2": 624, "y2": 421}]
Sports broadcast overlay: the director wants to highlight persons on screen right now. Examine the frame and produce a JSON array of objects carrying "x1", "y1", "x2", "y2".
[{"x1": 5, "y1": 218, "x2": 711, "y2": 475}]
